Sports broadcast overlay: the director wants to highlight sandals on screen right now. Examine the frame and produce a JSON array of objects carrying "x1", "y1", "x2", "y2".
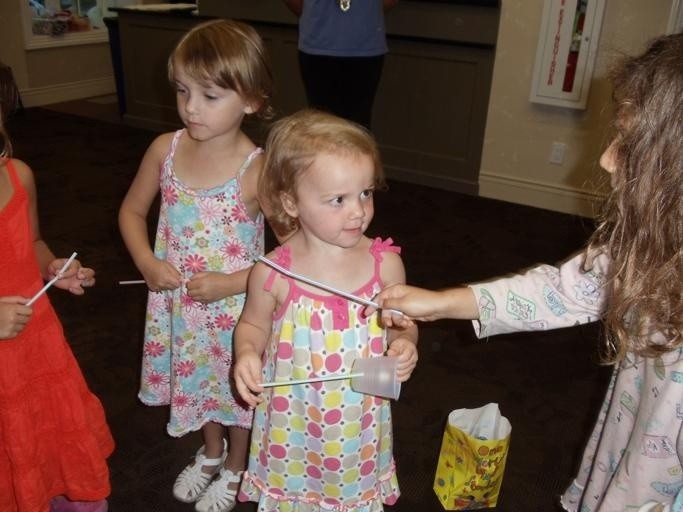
[
  {"x1": 195, "y1": 466, "x2": 246, "y2": 512},
  {"x1": 172, "y1": 435, "x2": 230, "y2": 504}
]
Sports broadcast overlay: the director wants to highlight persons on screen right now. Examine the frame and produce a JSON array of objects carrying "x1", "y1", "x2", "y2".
[
  {"x1": 296, "y1": 0, "x2": 389, "y2": 130},
  {"x1": 229, "y1": 107, "x2": 419, "y2": 511},
  {"x1": 115, "y1": 17, "x2": 300, "y2": 512},
  {"x1": 361, "y1": 31, "x2": 681, "y2": 511},
  {"x1": 0, "y1": 63, "x2": 116, "y2": 512}
]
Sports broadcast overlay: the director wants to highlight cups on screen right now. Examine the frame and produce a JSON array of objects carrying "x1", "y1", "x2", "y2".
[{"x1": 348, "y1": 355, "x2": 401, "y2": 398}]
